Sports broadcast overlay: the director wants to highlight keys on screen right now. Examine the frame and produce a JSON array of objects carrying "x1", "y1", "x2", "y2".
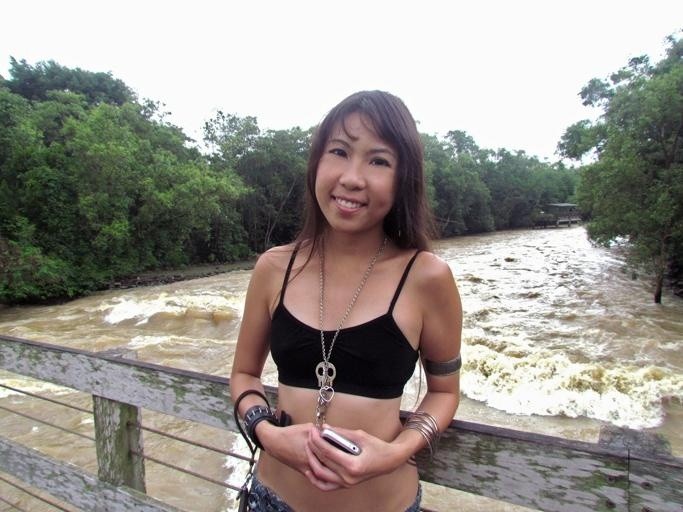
[{"x1": 236, "y1": 467, "x2": 253, "y2": 512}]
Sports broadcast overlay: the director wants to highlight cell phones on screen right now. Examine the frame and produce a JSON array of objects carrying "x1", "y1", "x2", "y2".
[{"x1": 318, "y1": 427, "x2": 361, "y2": 455}]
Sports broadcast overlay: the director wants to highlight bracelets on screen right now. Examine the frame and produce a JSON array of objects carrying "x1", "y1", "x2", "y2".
[
  {"x1": 397, "y1": 411, "x2": 440, "y2": 466},
  {"x1": 243, "y1": 405, "x2": 286, "y2": 450}
]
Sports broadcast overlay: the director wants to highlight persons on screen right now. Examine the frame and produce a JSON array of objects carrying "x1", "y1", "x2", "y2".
[{"x1": 230, "y1": 91, "x2": 463, "y2": 512}]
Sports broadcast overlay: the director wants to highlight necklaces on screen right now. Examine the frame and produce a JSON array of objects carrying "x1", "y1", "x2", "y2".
[{"x1": 314, "y1": 233, "x2": 390, "y2": 427}]
